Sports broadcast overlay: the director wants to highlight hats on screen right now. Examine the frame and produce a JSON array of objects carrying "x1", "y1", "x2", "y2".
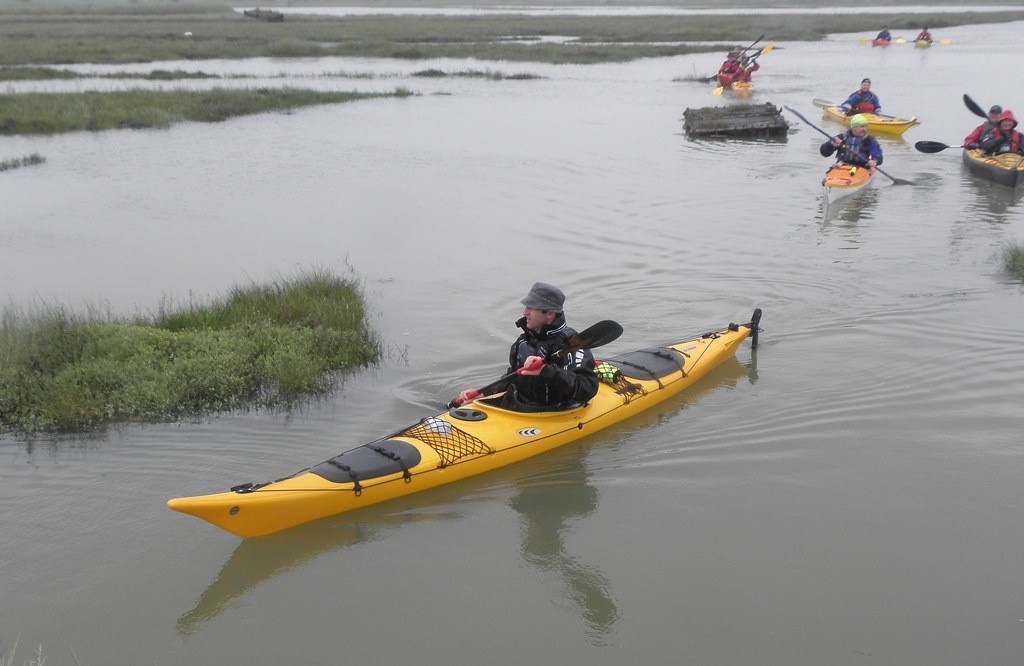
[
  {"x1": 850, "y1": 113, "x2": 869, "y2": 128},
  {"x1": 861, "y1": 78, "x2": 871, "y2": 83},
  {"x1": 731, "y1": 47, "x2": 741, "y2": 53},
  {"x1": 989, "y1": 105, "x2": 1002, "y2": 113},
  {"x1": 727, "y1": 52, "x2": 738, "y2": 58},
  {"x1": 519, "y1": 282, "x2": 566, "y2": 314}
]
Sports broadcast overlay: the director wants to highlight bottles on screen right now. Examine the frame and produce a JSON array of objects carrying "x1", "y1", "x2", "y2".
[
  {"x1": 420, "y1": 416, "x2": 452, "y2": 436},
  {"x1": 593, "y1": 359, "x2": 621, "y2": 383},
  {"x1": 849, "y1": 167, "x2": 857, "y2": 176}
]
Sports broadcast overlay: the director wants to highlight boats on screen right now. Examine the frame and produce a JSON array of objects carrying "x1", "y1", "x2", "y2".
[
  {"x1": 823, "y1": 158, "x2": 878, "y2": 204},
  {"x1": 165, "y1": 307, "x2": 762, "y2": 540},
  {"x1": 962, "y1": 147, "x2": 1024, "y2": 189},
  {"x1": 717, "y1": 74, "x2": 755, "y2": 97},
  {"x1": 872, "y1": 39, "x2": 890, "y2": 47},
  {"x1": 822, "y1": 106, "x2": 921, "y2": 136},
  {"x1": 914, "y1": 39, "x2": 929, "y2": 48}
]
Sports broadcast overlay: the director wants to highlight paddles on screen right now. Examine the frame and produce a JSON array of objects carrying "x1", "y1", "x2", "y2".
[
  {"x1": 784, "y1": 105, "x2": 915, "y2": 186},
  {"x1": 963, "y1": 94, "x2": 1024, "y2": 151},
  {"x1": 447, "y1": 319, "x2": 624, "y2": 408},
  {"x1": 812, "y1": 98, "x2": 921, "y2": 127},
  {"x1": 704, "y1": 34, "x2": 775, "y2": 96},
  {"x1": 896, "y1": 38, "x2": 954, "y2": 45},
  {"x1": 914, "y1": 139, "x2": 1024, "y2": 154},
  {"x1": 857, "y1": 36, "x2": 906, "y2": 45}
]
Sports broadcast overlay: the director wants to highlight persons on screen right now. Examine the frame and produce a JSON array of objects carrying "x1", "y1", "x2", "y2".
[
  {"x1": 717, "y1": 52, "x2": 739, "y2": 88},
  {"x1": 981, "y1": 110, "x2": 1024, "y2": 157},
  {"x1": 840, "y1": 78, "x2": 882, "y2": 116},
  {"x1": 915, "y1": 26, "x2": 932, "y2": 43},
  {"x1": 735, "y1": 52, "x2": 760, "y2": 81},
  {"x1": 458, "y1": 282, "x2": 599, "y2": 412},
  {"x1": 820, "y1": 114, "x2": 883, "y2": 170},
  {"x1": 964, "y1": 105, "x2": 1002, "y2": 149},
  {"x1": 876, "y1": 25, "x2": 891, "y2": 41}
]
